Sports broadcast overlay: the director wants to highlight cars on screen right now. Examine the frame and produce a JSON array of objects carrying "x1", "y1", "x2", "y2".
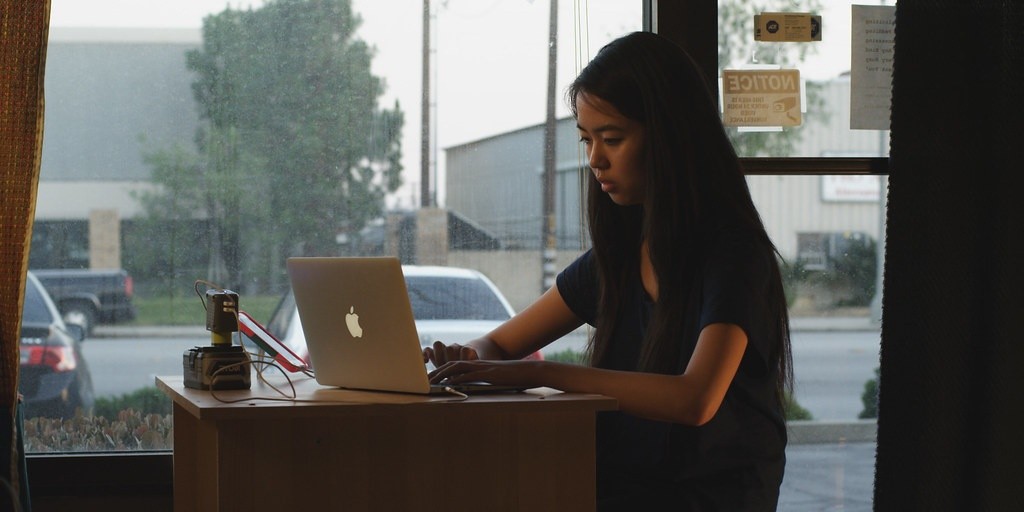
[
  {"x1": 18, "y1": 272, "x2": 98, "y2": 419},
  {"x1": 258, "y1": 267, "x2": 544, "y2": 395},
  {"x1": 333, "y1": 208, "x2": 500, "y2": 250}
]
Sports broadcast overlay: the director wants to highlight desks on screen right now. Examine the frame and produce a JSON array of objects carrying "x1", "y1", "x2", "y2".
[{"x1": 156, "y1": 371, "x2": 616, "y2": 512}]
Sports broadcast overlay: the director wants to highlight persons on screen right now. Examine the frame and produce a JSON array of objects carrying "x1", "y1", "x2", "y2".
[{"x1": 421, "y1": 30, "x2": 795, "y2": 512}]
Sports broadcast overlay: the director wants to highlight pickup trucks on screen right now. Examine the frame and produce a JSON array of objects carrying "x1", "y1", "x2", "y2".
[{"x1": 31, "y1": 270, "x2": 136, "y2": 337}]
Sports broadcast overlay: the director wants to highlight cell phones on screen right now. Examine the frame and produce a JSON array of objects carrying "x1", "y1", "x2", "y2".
[{"x1": 236, "y1": 312, "x2": 309, "y2": 373}]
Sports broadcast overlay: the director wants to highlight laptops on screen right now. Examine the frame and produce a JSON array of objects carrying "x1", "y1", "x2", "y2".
[{"x1": 286, "y1": 256, "x2": 525, "y2": 393}]
